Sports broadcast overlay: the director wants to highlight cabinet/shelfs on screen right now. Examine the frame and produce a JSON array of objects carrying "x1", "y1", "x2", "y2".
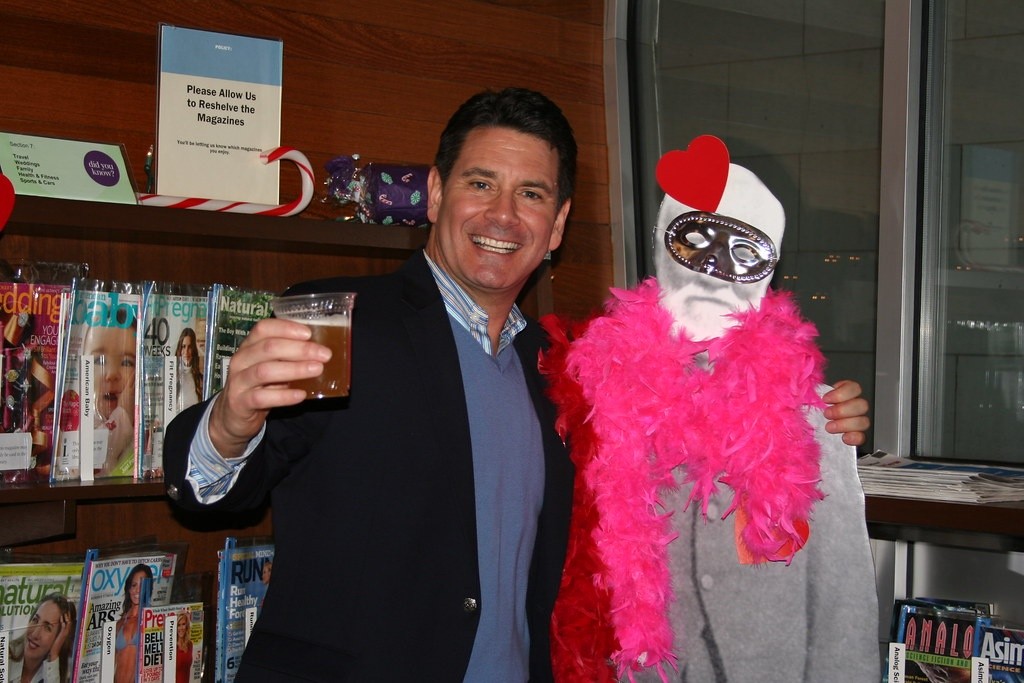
[{"x1": 1, "y1": 191, "x2": 436, "y2": 683}]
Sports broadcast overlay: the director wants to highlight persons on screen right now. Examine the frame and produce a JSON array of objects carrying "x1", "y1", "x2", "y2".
[
  {"x1": 9, "y1": 592, "x2": 77, "y2": 683},
  {"x1": 567, "y1": 136, "x2": 880, "y2": 683},
  {"x1": 161, "y1": 88, "x2": 869, "y2": 683},
  {"x1": 176, "y1": 614, "x2": 193, "y2": 683},
  {"x1": 262, "y1": 562, "x2": 272, "y2": 584},
  {"x1": 114, "y1": 564, "x2": 154, "y2": 683},
  {"x1": 174, "y1": 328, "x2": 203, "y2": 410},
  {"x1": 82, "y1": 307, "x2": 137, "y2": 476}
]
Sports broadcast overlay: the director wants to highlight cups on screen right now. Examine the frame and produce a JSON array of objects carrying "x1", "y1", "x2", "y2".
[{"x1": 271, "y1": 292, "x2": 356, "y2": 397}]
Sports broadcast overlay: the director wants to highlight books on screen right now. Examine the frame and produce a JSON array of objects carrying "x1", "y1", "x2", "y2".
[
  {"x1": 0, "y1": 277, "x2": 277, "y2": 683},
  {"x1": 882, "y1": 596, "x2": 1024, "y2": 683}
]
